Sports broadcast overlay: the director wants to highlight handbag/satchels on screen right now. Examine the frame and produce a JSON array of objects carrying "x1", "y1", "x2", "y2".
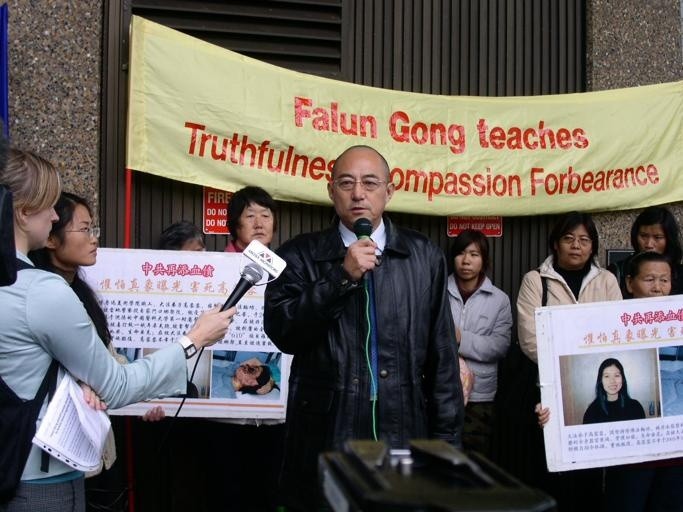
[
  {"x1": 492, "y1": 354, "x2": 541, "y2": 427},
  {"x1": 0, "y1": 373, "x2": 37, "y2": 506}
]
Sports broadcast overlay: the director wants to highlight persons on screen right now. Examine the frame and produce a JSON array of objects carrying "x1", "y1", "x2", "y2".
[
  {"x1": 606, "y1": 205, "x2": 683, "y2": 302},
  {"x1": 138, "y1": 220, "x2": 209, "y2": 509},
  {"x1": 24, "y1": 187, "x2": 121, "y2": 512},
  {"x1": 0, "y1": 146, "x2": 239, "y2": 512},
  {"x1": 581, "y1": 356, "x2": 646, "y2": 426},
  {"x1": 208, "y1": 352, "x2": 276, "y2": 402},
  {"x1": 220, "y1": 182, "x2": 278, "y2": 511},
  {"x1": 262, "y1": 143, "x2": 466, "y2": 512},
  {"x1": 515, "y1": 208, "x2": 624, "y2": 512},
  {"x1": 442, "y1": 228, "x2": 513, "y2": 461},
  {"x1": 533, "y1": 249, "x2": 683, "y2": 511}
]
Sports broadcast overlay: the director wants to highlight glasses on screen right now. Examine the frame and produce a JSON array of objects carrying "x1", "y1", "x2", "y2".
[
  {"x1": 556, "y1": 234, "x2": 594, "y2": 247},
  {"x1": 331, "y1": 178, "x2": 385, "y2": 192},
  {"x1": 62, "y1": 226, "x2": 101, "y2": 238}
]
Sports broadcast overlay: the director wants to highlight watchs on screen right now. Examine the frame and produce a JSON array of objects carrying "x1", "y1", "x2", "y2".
[{"x1": 175, "y1": 334, "x2": 198, "y2": 360}]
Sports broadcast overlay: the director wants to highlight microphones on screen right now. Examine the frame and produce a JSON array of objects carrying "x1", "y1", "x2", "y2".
[
  {"x1": 355, "y1": 216, "x2": 372, "y2": 282},
  {"x1": 219, "y1": 261, "x2": 264, "y2": 312}
]
